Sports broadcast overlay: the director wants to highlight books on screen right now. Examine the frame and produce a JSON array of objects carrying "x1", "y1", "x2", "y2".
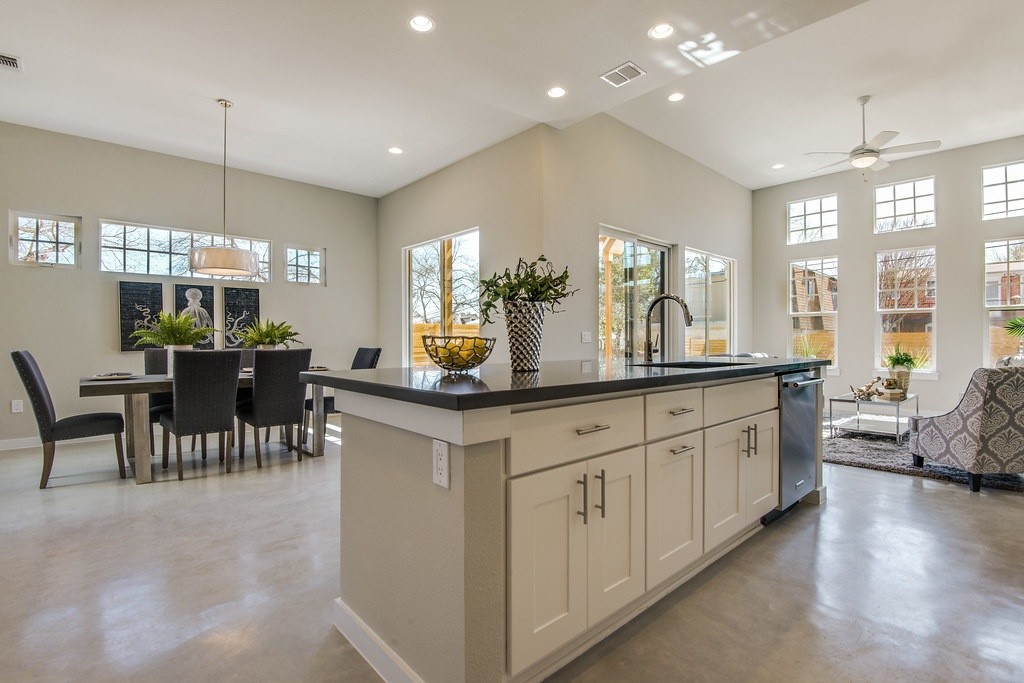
[{"x1": 878, "y1": 388, "x2": 905, "y2": 401}]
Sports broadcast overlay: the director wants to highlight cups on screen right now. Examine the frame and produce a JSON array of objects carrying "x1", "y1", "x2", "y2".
[{"x1": 882, "y1": 378, "x2": 898, "y2": 389}]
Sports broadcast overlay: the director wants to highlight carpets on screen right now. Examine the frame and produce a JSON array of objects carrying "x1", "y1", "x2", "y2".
[{"x1": 821, "y1": 406, "x2": 1023, "y2": 492}]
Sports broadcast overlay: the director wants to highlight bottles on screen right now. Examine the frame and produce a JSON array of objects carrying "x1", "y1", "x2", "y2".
[{"x1": 1014, "y1": 341, "x2": 1024, "y2": 366}]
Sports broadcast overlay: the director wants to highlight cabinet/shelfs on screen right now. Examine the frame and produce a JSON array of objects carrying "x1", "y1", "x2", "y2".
[
  {"x1": 702, "y1": 376, "x2": 785, "y2": 553},
  {"x1": 646, "y1": 390, "x2": 706, "y2": 589},
  {"x1": 504, "y1": 398, "x2": 645, "y2": 677}
]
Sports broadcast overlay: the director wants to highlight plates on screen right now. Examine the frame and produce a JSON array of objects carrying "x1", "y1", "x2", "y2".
[
  {"x1": 93, "y1": 375, "x2": 131, "y2": 378},
  {"x1": 309, "y1": 367, "x2": 329, "y2": 369}
]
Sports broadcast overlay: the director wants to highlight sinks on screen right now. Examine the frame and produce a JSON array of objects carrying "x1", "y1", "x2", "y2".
[{"x1": 621, "y1": 361, "x2": 759, "y2": 370}]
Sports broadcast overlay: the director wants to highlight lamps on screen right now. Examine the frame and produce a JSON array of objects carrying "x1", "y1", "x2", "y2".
[
  {"x1": 849, "y1": 149, "x2": 878, "y2": 169},
  {"x1": 190, "y1": 98, "x2": 259, "y2": 276}
]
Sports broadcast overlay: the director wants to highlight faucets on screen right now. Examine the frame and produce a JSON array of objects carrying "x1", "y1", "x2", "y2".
[{"x1": 643, "y1": 294, "x2": 693, "y2": 362}]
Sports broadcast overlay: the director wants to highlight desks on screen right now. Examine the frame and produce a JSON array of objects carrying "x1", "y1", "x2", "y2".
[{"x1": 78, "y1": 364, "x2": 331, "y2": 487}]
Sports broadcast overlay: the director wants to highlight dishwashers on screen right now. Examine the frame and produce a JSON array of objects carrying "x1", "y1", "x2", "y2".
[{"x1": 761, "y1": 370, "x2": 825, "y2": 527}]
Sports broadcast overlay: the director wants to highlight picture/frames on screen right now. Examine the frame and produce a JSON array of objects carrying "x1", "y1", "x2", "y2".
[
  {"x1": 171, "y1": 282, "x2": 216, "y2": 350},
  {"x1": 219, "y1": 288, "x2": 260, "y2": 347},
  {"x1": 119, "y1": 280, "x2": 165, "y2": 351}
]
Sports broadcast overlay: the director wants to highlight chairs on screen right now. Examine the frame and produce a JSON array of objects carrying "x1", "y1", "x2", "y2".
[
  {"x1": 908, "y1": 367, "x2": 1023, "y2": 492},
  {"x1": 303, "y1": 346, "x2": 383, "y2": 447},
  {"x1": 145, "y1": 346, "x2": 171, "y2": 458},
  {"x1": 11, "y1": 349, "x2": 128, "y2": 490},
  {"x1": 237, "y1": 347, "x2": 312, "y2": 469},
  {"x1": 160, "y1": 349, "x2": 243, "y2": 481},
  {"x1": 229, "y1": 347, "x2": 272, "y2": 450}
]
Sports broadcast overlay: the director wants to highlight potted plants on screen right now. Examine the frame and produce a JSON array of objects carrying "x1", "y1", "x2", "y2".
[
  {"x1": 885, "y1": 343, "x2": 916, "y2": 398},
  {"x1": 129, "y1": 311, "x2": 221, "y2": 379},
  {"x1": 478, "y1": 255, "x2": 575, "y2": 376},
  {"x1": 235, "y1": 317, "x2": 304, "y2": 350}
]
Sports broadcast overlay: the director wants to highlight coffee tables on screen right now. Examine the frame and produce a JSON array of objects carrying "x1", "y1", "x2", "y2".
[{"x1": 828, "y1": 386, "x2": 919, "y2": 444}]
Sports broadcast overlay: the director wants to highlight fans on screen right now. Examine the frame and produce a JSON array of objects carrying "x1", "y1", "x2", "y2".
[{"x1": 801, "y1": 100, "x2": 942, "y2": 178}]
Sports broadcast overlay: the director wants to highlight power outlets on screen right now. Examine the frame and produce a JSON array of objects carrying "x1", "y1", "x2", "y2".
[
  {"x1": 11, "y1": 399, "x2": 24, "y2": 414},
  {"x1": 431, "y1": 439, "x2": 449, "y2": 489}
]
